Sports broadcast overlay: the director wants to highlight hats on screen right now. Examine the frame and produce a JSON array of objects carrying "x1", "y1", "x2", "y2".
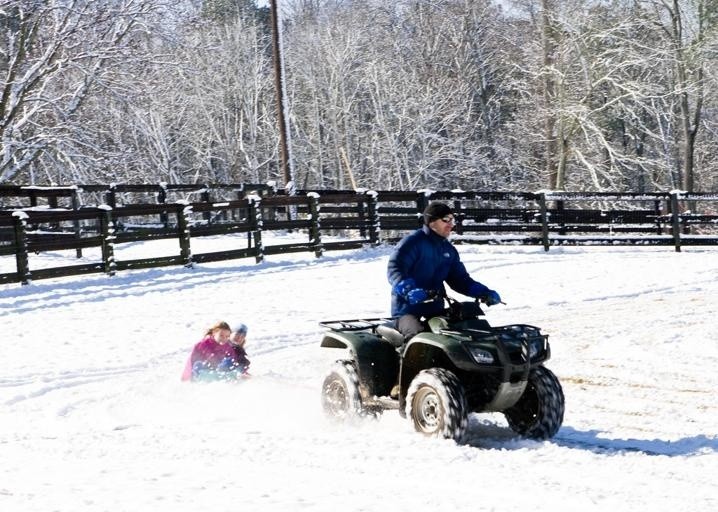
[{"x1": 423, "y1": 202, "x2": 451, "y2": 225}]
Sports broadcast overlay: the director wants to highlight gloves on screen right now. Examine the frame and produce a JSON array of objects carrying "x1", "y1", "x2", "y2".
[
  {"x1": 469, "y1": 283, "x2": 500, "y2": 307},
  {"x1": 394, "y1": 279, "x2": 426, "y2": 304}
]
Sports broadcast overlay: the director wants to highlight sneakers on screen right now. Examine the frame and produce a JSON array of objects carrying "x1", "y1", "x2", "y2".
[{"x1": 390, "y1": 384, "x2": 399, "y2": 399}]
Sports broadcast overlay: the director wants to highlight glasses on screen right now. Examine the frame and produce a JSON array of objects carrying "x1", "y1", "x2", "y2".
[{"x1": 442, "y1": 216, "x2": 453, "y2": 223}]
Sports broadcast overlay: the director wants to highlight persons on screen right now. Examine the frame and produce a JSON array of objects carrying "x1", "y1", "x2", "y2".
[
  {"x1": 229, "y1": 324, "x2": 251, "y2": 367},
  {"x1": 387, "y1": 202, "x2": 501, "y2": 400},
  {"x1": 181, "y1": 321, "x2": 237, "y2": 382}
]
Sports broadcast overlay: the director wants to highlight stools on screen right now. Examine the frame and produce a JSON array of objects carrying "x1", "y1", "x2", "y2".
[{"x1": 376, "y1": 319, "x2": 406, "y2": 349}]
